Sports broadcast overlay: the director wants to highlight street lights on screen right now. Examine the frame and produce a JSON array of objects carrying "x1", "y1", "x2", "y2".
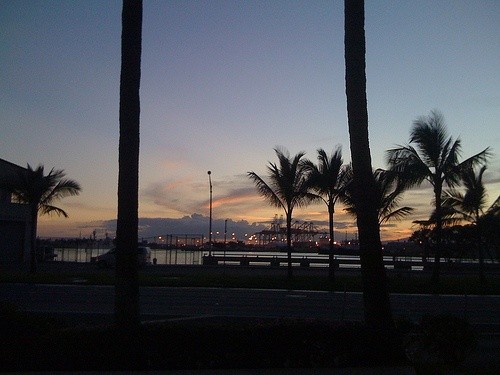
[
  {"x1": 207, "y1": 171, "x2": 213, "y2": 256},
  {"x1": 225, "y1": 219, "x2": 227, "y2": 242}
]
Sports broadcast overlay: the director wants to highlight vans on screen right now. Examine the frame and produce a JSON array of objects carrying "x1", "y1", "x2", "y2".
[{"x1": 97, "y1": 246, "x2": 152, "y2": 269}]
